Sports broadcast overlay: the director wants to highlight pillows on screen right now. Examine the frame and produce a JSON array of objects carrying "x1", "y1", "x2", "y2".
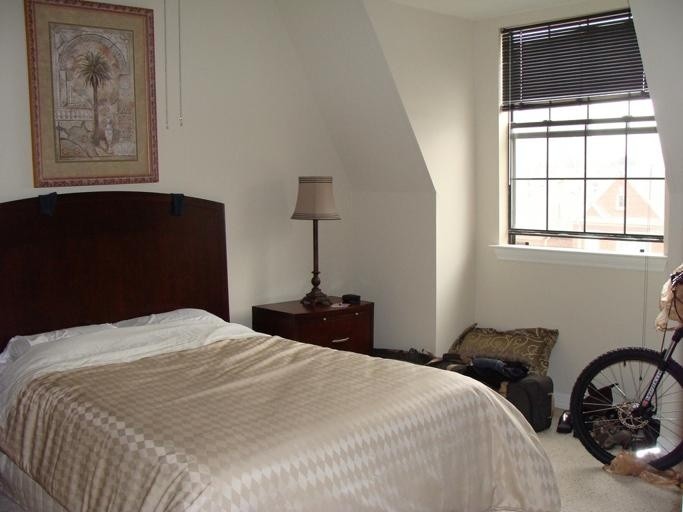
[{"x1": 448, "y1": 322, "x2": 559, "y2": 376}]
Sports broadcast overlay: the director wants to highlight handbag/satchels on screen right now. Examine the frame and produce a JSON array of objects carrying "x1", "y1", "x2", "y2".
[
  {"x1": 581, "y1": 382, "x2": 613, "y2": 417},
  {"x1": 372, "y1": 345, "x2": 432, "y2": 364}
]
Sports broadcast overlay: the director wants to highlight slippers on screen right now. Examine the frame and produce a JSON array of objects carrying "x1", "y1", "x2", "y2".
[{"x1": 557, "y1": 410, "x2": 573, "y2": 432}]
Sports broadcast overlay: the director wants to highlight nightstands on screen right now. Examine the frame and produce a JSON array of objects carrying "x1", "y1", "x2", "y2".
[{"x1": 252, "y1": 295, "x2": 374, "y2": 355}]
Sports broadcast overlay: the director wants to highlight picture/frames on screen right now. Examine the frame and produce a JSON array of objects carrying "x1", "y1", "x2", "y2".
[{"x1": 24, "y1": 0, "x2": 159, "y2": 187}]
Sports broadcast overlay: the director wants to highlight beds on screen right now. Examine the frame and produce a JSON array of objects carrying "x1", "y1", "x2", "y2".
[{"x1": 1, "y1": 192, "x2": 562, "y2": 512}]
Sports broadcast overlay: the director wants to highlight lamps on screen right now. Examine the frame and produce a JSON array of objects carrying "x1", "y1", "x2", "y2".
[{"x1": 291, "y1": 176, "x2": 342, "y2": 307}]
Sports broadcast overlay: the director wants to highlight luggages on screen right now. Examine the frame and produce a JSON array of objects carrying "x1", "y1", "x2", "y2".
[{"x1": 430, "y1": 361, "x2": 553, "y2": 432}]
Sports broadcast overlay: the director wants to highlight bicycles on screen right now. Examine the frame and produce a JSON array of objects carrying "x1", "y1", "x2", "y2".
[{"x1": 567, "y1": 273, "x2": 683, "y2": 474}]
